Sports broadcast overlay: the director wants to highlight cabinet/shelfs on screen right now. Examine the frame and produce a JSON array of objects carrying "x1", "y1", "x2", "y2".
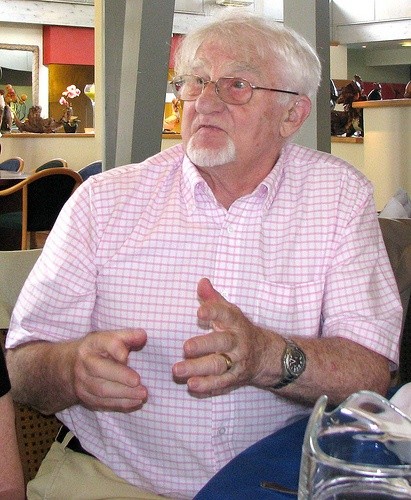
[{"x1": 0, "y1": 97, "x2": 411, "y2": 214}]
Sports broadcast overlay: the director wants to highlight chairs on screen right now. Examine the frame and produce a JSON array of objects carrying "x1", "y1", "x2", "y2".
[{"x1": 0, "y1": 144, "x2": 102, "y2": 250}]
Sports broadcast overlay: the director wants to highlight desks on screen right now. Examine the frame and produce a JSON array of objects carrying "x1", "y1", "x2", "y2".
[
  {"x1": 194, "y1": 383, "x2": 411, "y2": 500},
  {"x1": 0, "y1": 170, "x2": 36, "y2": 185}
]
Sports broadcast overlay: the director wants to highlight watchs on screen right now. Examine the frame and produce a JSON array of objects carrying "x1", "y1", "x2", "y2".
[{"x1": 262, "y1": 332, "x2": 306, "y2": 391}]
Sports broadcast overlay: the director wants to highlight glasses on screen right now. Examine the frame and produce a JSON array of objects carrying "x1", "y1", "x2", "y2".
[{"x1": 169, "y1": 72, "x2": 299, "y2": 105}]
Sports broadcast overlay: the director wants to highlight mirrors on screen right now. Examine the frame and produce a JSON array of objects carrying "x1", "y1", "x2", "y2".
[{"x1": 0, "y1": 43, "x2": 39, "y2": 129}]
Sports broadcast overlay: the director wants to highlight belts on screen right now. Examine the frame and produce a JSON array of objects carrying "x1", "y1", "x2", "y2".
[{"x1": 54, "y1": 425, "x2": 97, "y2": 459}]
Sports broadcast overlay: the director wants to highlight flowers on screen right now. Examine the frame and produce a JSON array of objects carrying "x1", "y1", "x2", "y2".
[{"x1": 59, "y1": 85, "x2": 81, "y2": 105}]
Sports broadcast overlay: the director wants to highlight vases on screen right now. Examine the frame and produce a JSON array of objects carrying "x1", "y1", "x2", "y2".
[{"x1": 63, "y1": 107, "x2": 78, "y2": 133}]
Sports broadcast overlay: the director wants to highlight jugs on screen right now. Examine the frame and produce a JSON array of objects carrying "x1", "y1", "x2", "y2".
[{"x1": 295, "y1": 391, "x2": 411, "y2": 500}]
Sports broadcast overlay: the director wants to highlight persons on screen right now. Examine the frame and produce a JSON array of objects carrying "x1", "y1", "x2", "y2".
[{"x1": 4, "y1": 11, "x2": 403, "y2": 500}]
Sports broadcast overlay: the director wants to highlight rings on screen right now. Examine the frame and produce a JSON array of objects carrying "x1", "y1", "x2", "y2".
[{"x1": 217, "y1": 353, "x2": 231, "y2": 372}]
